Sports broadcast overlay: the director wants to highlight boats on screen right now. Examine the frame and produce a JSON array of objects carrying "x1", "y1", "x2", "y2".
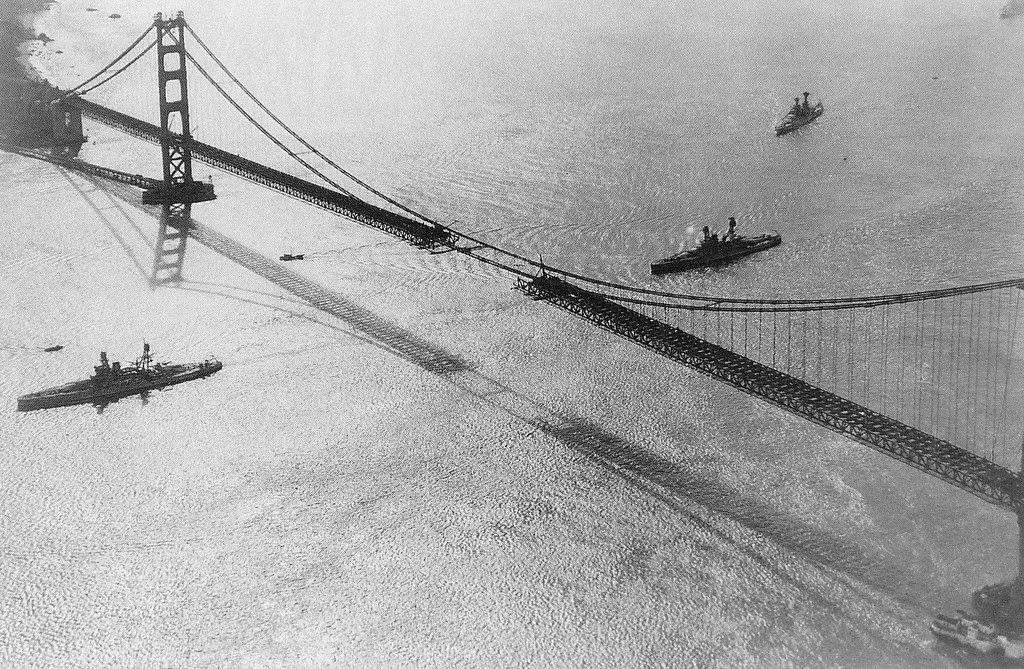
[
  {"x1": 280, "y1": 254, "x2": 302, "y2": 260},
  {"x1": 15, "y1": 341, "x2": 223, "y2": 413},
  {"x1": 651, "y1": 215, "x2": 780, "y2": 273},
  {"x1": 773, "y1": 90, "x2": 825, "y2": 132}
]
